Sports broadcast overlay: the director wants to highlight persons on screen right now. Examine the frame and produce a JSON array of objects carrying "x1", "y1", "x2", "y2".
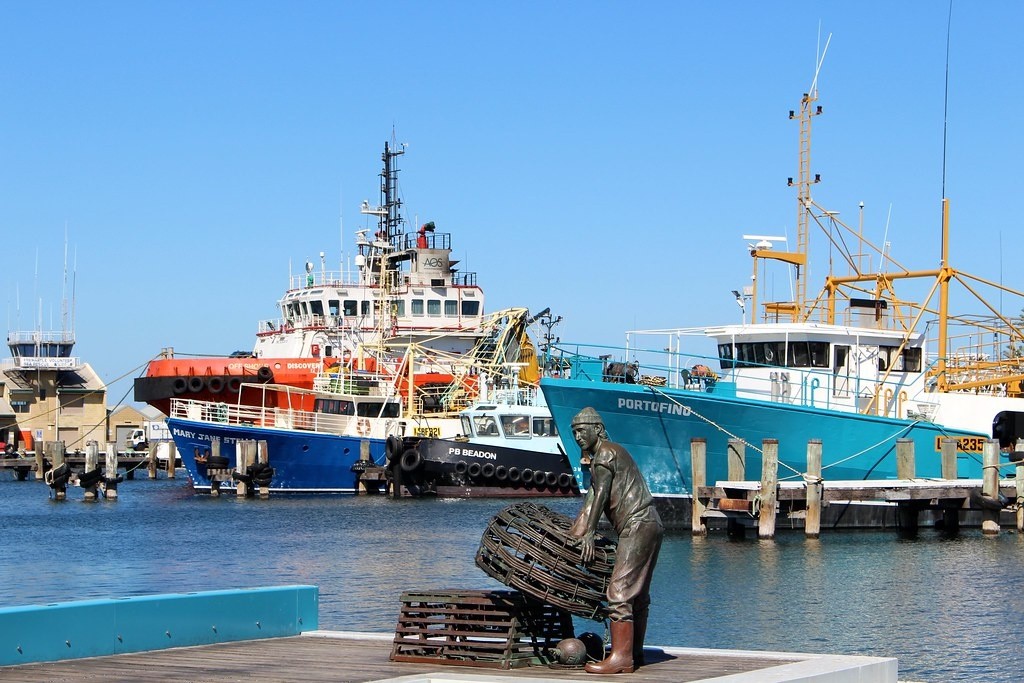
[{"x1": 571, "y1": 406, "x2": 664, "y2": 674}]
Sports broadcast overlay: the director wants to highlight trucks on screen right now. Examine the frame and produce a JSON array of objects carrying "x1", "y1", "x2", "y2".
[{"x1": 127, "y1": 420, "x2": 175, "y2": 452}]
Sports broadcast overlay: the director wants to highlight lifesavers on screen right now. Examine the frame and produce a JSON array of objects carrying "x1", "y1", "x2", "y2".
[
  {"x1": 454, "y1": 460, "x2": 579, "y2": 489},
  {"x1": 171, "y1": 365, "x2": 273, "y2": 395},
  {"x1": 385, "y1": 434, "x2": 422, "y2": 472},
  {"x1": 970, "y1": 486, "x2": 1011, "y2": 512},
  {"x1": 356, "y1": 419, "x2": 371, "y2": 434}
]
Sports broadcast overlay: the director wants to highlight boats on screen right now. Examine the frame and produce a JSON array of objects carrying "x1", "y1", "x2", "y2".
[
  {"x1": 164, "y1": 253, "x2": 563, "y2": 498},
  {"x1": 385, "y1": 308, "x2": 583, "y2": 510},
  {"x1": 133, "y1": 120, "x2": 571, "y2": 429},
  {"x1": 541, "y1": 19, "x2": 1024, "y2": 530}
]
